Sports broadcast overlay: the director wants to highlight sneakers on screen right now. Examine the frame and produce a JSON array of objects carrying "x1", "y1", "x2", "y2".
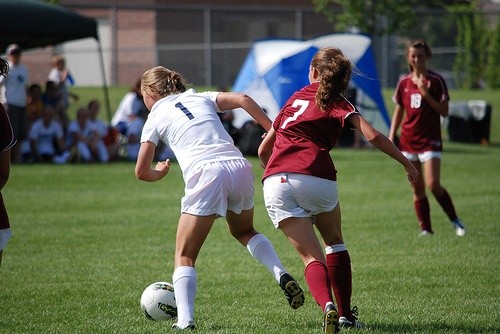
[
  {"x1": 172, "y1": 324, "x2": 195, "y2": 330},
  {"x1": 278, "y1": 273, "x2": 305, "y2": 309},
  {"x1": 338, "y1": 306, "x2": 363, "y2": 328},
  {"x1": 323, "y1": 304, "x2": 340, "y2": 334}
]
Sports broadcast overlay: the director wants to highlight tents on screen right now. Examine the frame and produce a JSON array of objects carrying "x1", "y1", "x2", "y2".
[
  {"x1": 0, "y1": 0, "x2": 112, "y2": 129},
  {"x1": 231, "y1": 34, "x2": 392, "y2": 149}
]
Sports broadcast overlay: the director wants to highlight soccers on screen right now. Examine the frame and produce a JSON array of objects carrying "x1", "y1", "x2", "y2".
[{"x1": 140, "y1": 281, "x2": 178, "y2": 322}]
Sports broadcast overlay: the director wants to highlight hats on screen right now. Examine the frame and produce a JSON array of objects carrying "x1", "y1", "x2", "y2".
[{"x1": 8, "y1": 44, "x2": 20, "y2": 55}]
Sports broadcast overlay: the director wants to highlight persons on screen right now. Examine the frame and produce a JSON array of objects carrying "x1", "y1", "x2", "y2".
[
  {"x1": 134, "y1": 65, "x2": 306, "y2": 330},
  {"x1": 0, "y1": 58, "x2": 17, "y2": 273},
  {"x1": 18, "y1": 55, "x2": 178, "y2": 163},
  {"x1": 214, "y1": 85, "x2": 270, "y2": 158},
  {"x1": 389, "y1": 40, "x2": 467, "y2": 237},
  {"x1": 258, "y1": 47, "x2": 419, "y2": 334},
  {"x1": 0, "y1": 44, "x2": 29, "y2": 164}
]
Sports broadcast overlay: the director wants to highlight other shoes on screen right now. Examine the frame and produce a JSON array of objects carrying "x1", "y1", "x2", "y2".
[
  {"x1": 452, "y1": 220, "x2": 467, "y2": 237},
  {"x1": 421, "y1": 230, "x2": 430, "y2": 236}
]
